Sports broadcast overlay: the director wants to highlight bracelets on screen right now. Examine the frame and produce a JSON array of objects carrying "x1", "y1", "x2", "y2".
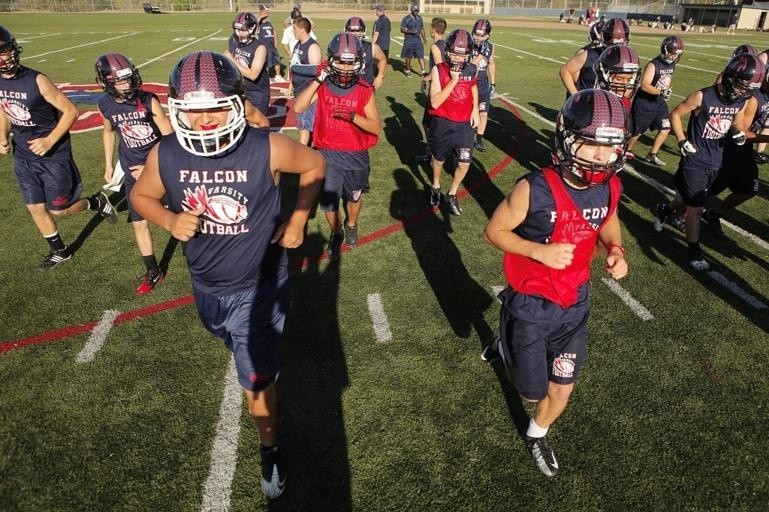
[{"x1": 611, "y1": 245, "x2": 627, "y2": 255}]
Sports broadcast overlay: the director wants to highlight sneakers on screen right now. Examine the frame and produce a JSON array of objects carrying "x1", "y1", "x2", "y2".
[
  {"x1": 524, "y1": 434, "x2": 558, "y2": 477},
  {"x1": 480, "y1": 335, "x2": 500, "y2": 361},
  {"x1": 415, "y1": 141, "x2": 486, "y2": 216},
  {"x1": 327, "y1": 230, "x2": 343, "y2": 254},
  {"x1": 649, "y1": 201, "x2": 721, "y2": 270},
  {"x1": 275, "y1": 75, "x2": 286, "y2": 82},
  {"x1": 754, "y1": 153, "x2": 768, "y2": 163},
  {"x1": 136, "y1": 268, "x2": 163, "y2": 294},
  {"x1": 646, "y1": 152, "x2": 665, "y2": 166},
  {"x1": 260, "y1": 444, "x2": 287, "y2": 499},
  {"x1": 344, "y1": 222, "x2": 357, "y2": 247},
  {"x1": 404, "y1": 69, "x2": 411, "y2": 75},
  {"x1": 93, "y1": 191, "x2": 118, "y2": 224},
  {"x1": 36, "y1": 245, "x2": 72, "y2": 270},
  {"x1": 422, "y1": 70, "x2": 427, "y2": 76}
]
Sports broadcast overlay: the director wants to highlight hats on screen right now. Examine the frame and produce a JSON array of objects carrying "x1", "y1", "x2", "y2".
[
  {"x1": 291, "y1": 11, "x2": 301, "y2": 19},
  {"x1": 260, "y1": 5, "x2": 269, "y2": 11},
  {"x1": 374, "y1": 5, "x2": 384, "y2": 11}
]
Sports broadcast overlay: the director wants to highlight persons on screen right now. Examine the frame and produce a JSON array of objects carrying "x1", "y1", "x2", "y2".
[
  {"x1": 0, "y1": 27, "x2": 118, "y2": 272},
  {"x1": 629, "y1": 38, "x2": 684, "y2": 168},
  {"x1": 225, "y1": 3, "x2": 391, "y2": 145},
  {"x1": 648, "y1": 43, "x2": 765, "y2": 271},
  {"x1": 400, "y1": 5, "x2": 494, "y2": 216},
  {"x1": 293, "y1": 32, "x2": 380, "y2": 256},
  {"x1": 593, "y1": 44, "x2": 639, "y2": 127},
  {"x1": 95, "y1": 55, "x2": 172, "y2": 295},
  {"x1": 480, "y1": 92, "x2": 629, "y2": 479},
  {"x1": 131, "y1": 53, "x2": 329, "y2": 498},
  {"x1": 565, "y1": 22, "x2": 605, "y2": 101},
  {"x1": 557, "y1": 3, "x2": 738, "y2": 29},
  {"x1": 560, "y1": 19, "x2": 640, "y2": 100}
]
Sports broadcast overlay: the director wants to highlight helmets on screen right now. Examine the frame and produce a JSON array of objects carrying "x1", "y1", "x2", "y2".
[
  {"x1": 410, "y1": 6, "x2": 419, "y2": 12},
  {"x1": 715, "y1": 46, "x2": 767, "y2": 104},
  {"x1": 345, "y1": 17, "x2": 365, "y2": 40},
  {"x1": 94, "y1": 53, "x2": 142, "y2": 99},
  {"x1": 472, "y1": 19, "x2": 491, "y2": 43},
  {"x1": 444, "y1": 29, "x2": 473, "y2": 64},
  {"x1": 327, "y1": 33, "x2": 363, "y2": 89},
  {"x1": 232, "y1": 12, "x2": 258, "y2": 44},
  {"x1": 589, "y1": 19, "x2": 684, "y2": 104},
  {"x1": 167, "y1": 52, "x2": 247, "y2": 156},
  {"x1": 0, "y1": 26, "x2": 20, "y2": 72},
  {"x1": 550, "y1": 89, "x2": 630, "y2": 185}
]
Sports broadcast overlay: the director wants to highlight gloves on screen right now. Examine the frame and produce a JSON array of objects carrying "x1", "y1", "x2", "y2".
[
  {"x1": 315, "y1": 61, "x2": 331, "y2": 81},
  {"x1": 732, "y1": 131, "x2": 746, "y2": 146},
  {"x1": 490, "y1": 84, "x2": 495, "y2": 96},
  {"x1": 478, "y1": 41, "x2": 487, "y2": 54},
  {"x1": 678, "y1": 140, "x2": 698, "y2": 156},
  {"x1": 660, "y1": 89, "x2": 672, "y2": 97},
  {"x1": 330, "y1": 105, "x2": 355, "y2": 123}
]
